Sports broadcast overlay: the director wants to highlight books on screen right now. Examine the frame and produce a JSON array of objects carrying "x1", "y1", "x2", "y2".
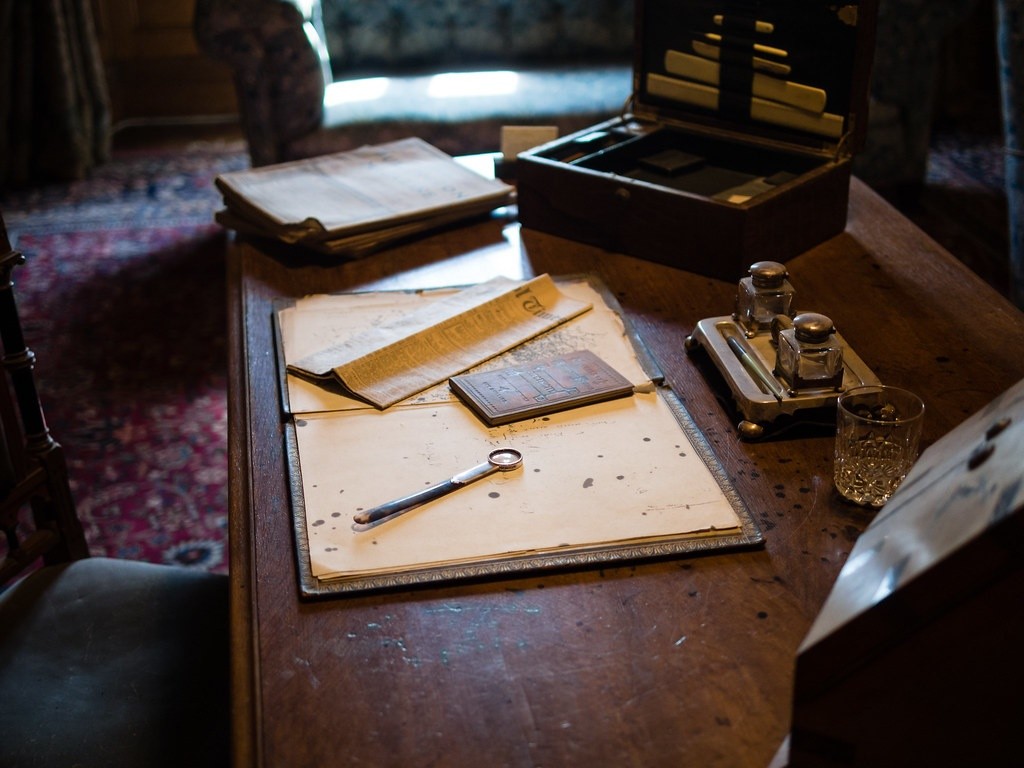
[
  {"x1": 273, "y1": 272, "x2": 767, "y2": 592},
  {"x1": 449, "y1": 350, "x2": 635, "y2": 427},
  {"x1": 214, "y1": 135, "x2": 517, "y2": 261}
]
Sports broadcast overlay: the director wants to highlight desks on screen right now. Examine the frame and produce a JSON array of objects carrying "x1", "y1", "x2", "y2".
[{"x1": 231, "y1": 153, "x2": 1024, "y2": 768}]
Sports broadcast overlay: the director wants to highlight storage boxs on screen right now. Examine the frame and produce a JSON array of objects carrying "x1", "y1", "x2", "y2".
[{"x1": 516, "y1": 0, "x2": 880, "y2": 287}]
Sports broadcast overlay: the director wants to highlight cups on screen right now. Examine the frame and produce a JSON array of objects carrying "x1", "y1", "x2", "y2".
[{"x1": 834, "y1": 387, "x2": 925, "y2": 508}]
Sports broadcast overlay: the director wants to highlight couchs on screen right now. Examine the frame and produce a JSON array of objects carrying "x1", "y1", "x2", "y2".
[{"x1": 191, "y1": 0, "x2": 974, "y2": 189}]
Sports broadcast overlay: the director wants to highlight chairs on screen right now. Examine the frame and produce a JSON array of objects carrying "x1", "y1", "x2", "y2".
[{"x1": 0, "y1": 206, "x2": 235, "y2": 767}]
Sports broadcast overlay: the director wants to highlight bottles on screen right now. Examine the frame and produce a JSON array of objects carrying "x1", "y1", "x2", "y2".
[
  {"x1": 779, "y1": 313, "x2": 841, "y2": 378},
  {"x1": 737, "y1": 262, "x2": 794, "y2": 322}
]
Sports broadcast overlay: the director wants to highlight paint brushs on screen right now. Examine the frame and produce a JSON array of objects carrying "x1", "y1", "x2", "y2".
[{"x1": 719, "y1": 326, "x2": 784, "y2": 402}]
